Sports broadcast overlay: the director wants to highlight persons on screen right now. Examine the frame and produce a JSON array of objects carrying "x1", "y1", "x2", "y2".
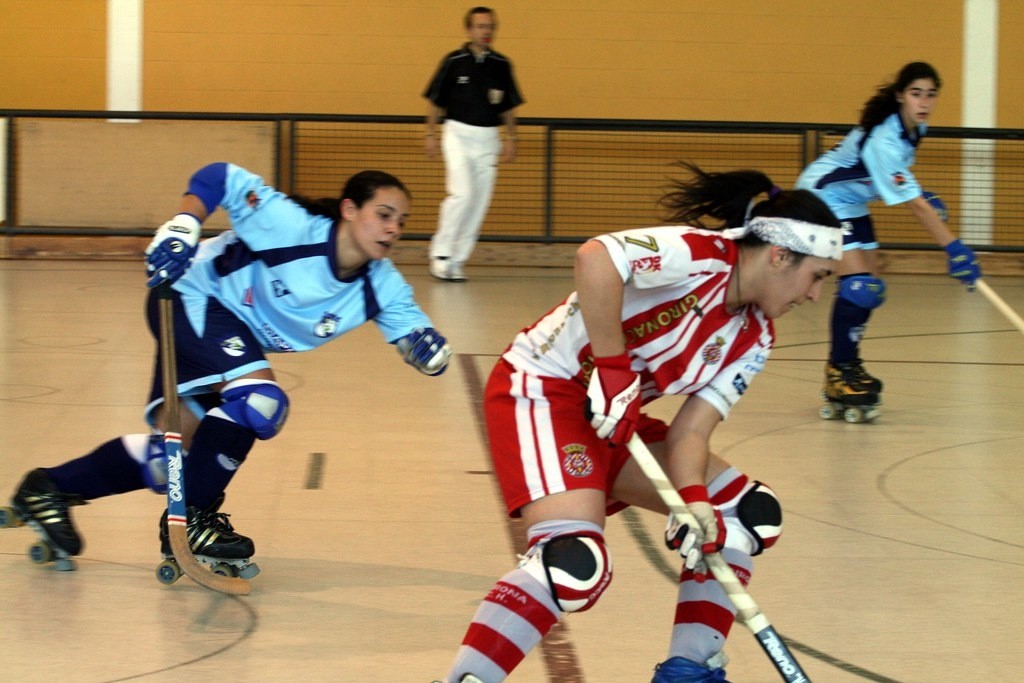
[
  {"x1": 421, "y1": 7, "x2": 526, "y2": 281},
  {"x1": 439, "y1": 160, "x2": 844, "y2": 683},
  {"x1": 0, "y1": 161, "x2": 451, "y2": 584},
  {"x1": 793, "y1": 61, "x2": 983, "y2": 424}
]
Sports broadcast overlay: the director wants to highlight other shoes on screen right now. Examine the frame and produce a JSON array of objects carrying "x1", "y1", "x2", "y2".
[
  {"x1": 650, "y1": 651, "x2": 734, "y2": 683},
  {"x1": 427, "y1": 256, "x2": 467, "y2": 280}
]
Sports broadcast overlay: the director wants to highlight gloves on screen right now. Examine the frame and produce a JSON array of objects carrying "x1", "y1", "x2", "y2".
[
  {"x1": 396, "y1": 325, "x2": 454, "y2": 376},
  {"x1": 664, "y1": 484, "x2": 727, "y2": 584},
  {"x1": 582, "y1": 351, "x2": 643, "y2": 448},
  {"x1": 943, "y1": 238, "x2": 982, "y2": 292},
  {"x1": 921, "y1": 191, "x2": 948, "y2": 220},
  {"x1": 143, "y1": 212, "x2": 203, "y2": 290}
]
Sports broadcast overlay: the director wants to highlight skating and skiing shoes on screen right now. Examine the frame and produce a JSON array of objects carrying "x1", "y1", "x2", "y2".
[
  {"x1": 816, "y1": 347, "x2": 883, "y2": 423},
  {"x1": 156, "y1": 490, "x2": 260, "y2": 584},
  {"x1": 0, "y1": 468, "x2": 88, "y2": 571}
]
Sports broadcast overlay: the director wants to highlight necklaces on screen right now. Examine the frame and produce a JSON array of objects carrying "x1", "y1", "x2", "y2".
[{"x1": 735, "y1": 262, "x2": 750, "y2": 332}]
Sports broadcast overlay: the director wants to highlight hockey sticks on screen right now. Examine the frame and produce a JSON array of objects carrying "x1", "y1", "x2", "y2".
[
  {"x1": 974, "y1": 277, "x2": 1024, "y2": 335},
  {"x1": 624, "y1": 431, "x2": 815, "y2": 683},
  {"x1": 156, "y1": 279, "x2": 256, "y2": 599}
]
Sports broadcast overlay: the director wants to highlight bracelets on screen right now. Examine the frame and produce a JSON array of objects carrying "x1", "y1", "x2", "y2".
[{"x1": 506, "y1": 136, "x2": 517, "y2": 141}]
